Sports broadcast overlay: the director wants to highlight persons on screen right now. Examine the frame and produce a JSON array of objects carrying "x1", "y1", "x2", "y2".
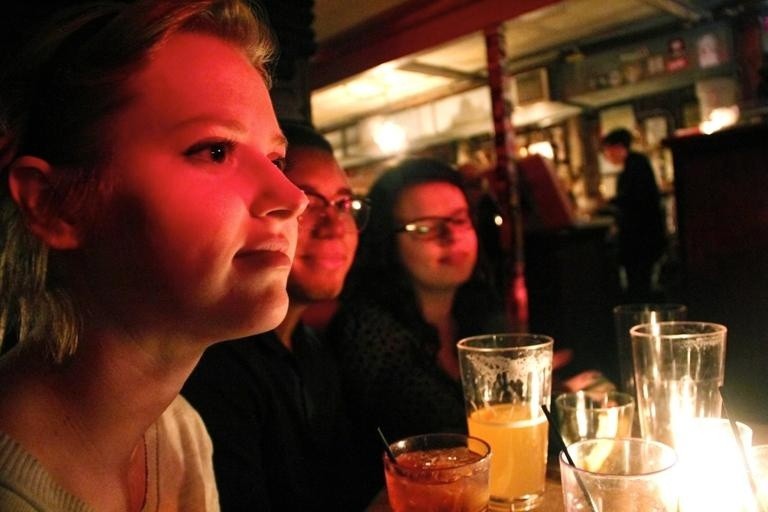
[
  {"x1": 577, "y1": 126, "x2": 672, "y2": 303},
  {"x1": 318, "y1": 155, "x2": 620, "y2": 486},
  {"x1": 0, "y1": 0, "x2": 310, "y2": 511},
  {"x1": 182, "y1": 114, "x2": 380, "y2": 512}
]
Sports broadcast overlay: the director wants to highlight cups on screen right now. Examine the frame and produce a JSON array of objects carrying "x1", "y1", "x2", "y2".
[
  {"x1": 611, "y1": 301, "x2": 687, "y2": 429},
  {"x1": 558, "y1": 437, "x2": 680, "y2": 512},
  {"x1": 629, "y1": 321, "x2": 728, "y2": 447},
  {"x1": 555, "y1": 390, "x2": 635, "y2": 496},
  {"x1": 456, "y1": 332, "x2": 554, "y2": 512},
  {"x1": 383, "y1": 433, "x2": 492, "y2": 512}
]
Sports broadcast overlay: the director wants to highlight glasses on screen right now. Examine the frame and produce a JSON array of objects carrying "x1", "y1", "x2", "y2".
[
  {"x1": 297, "y1": 192, "x2": 374, "y2": 236},
  {"x1": 395, "y1": 208, "x2": 479, "y2": 241}
]
